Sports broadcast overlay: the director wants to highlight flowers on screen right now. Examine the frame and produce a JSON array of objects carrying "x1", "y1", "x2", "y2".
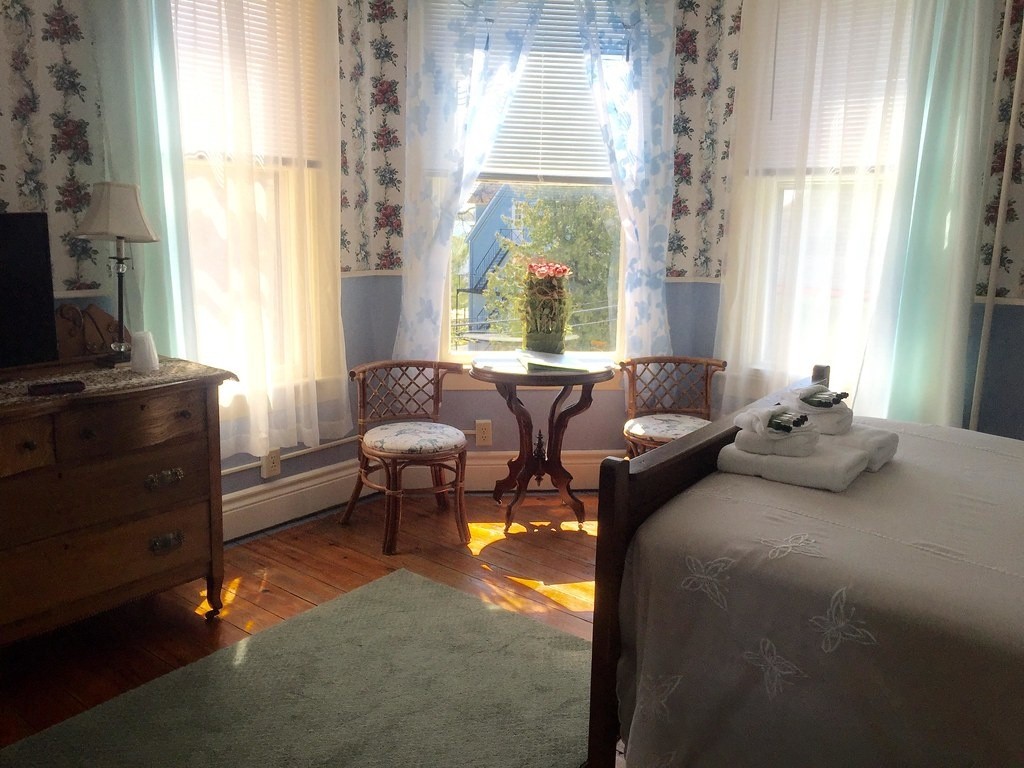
[{"x1": 522, "y1": 262, "x2": 574, "y2": 334}]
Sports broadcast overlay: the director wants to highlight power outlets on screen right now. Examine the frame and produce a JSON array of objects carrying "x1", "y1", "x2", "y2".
[{"x1": 475, "y1": 419, "x2": 493, "y2": 447}]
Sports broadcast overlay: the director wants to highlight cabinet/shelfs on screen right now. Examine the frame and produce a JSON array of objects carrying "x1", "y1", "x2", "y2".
[{"x1": 2, "y1": 349, "x2": 238, "y2": 652}]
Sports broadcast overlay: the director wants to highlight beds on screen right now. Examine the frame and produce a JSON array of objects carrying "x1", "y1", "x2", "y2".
[{"x1": 582, "y1": 366, "x2": 1022, "y2": 768}]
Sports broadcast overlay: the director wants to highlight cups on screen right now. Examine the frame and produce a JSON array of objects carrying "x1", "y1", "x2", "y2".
[{"x1": 128, "y1": 332, "x2": 159, "y2": 372}]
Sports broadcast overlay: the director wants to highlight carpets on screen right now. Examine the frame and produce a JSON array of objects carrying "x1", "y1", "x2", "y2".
[{"x1": 4, "y1": 565, "x2": 591, "y2": 768}]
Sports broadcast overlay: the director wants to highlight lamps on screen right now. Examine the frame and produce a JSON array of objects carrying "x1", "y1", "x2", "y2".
[{"x1": 71, "y1": 183, "x2": 161, "y2": 370}]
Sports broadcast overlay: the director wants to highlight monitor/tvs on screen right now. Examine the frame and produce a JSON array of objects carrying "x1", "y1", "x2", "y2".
[{"x1": 0, "y1": 212, "x2": 57, "y2": 368}]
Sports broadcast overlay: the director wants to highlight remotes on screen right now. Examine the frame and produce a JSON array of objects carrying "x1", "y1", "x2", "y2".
[{"x1": 29, "y1": 381, "x2": 85, "y2": 397}]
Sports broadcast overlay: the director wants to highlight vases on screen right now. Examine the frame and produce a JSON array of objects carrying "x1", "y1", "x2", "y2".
[{"x1": 524, "y1": 331, "x2": 566, "y2": 353}]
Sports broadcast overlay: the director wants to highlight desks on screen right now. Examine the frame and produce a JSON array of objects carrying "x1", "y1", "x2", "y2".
[{"x1": 469, "y1": 350, "x2": 616, "y2": 535}]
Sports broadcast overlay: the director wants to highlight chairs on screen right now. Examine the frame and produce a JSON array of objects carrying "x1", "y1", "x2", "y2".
[
  {"x1": 340, "y1": 360, "x2": 468, "y2": 555},
  {"x1": 619, "y1": 356, "x2": 727, "y2": 460}
]
